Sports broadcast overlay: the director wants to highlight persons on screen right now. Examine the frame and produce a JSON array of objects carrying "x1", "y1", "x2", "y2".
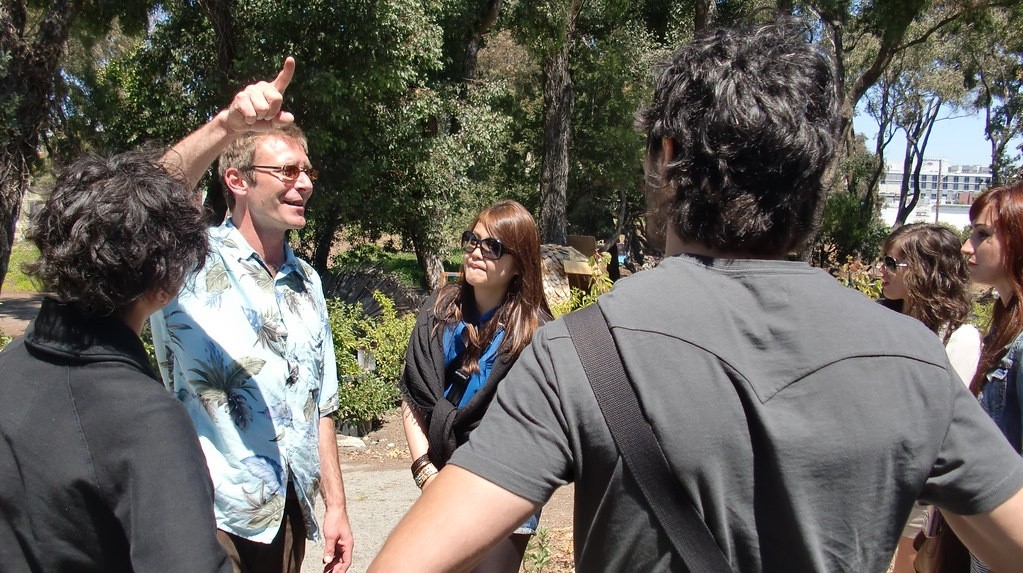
[
  {"x1": 361, "y1": 20, "x2": 1023, "y2": 573},
  {"x1": 122, "y1": 56, "x2": 354, "y2": 573},
  {"x1": 0, "y1": 140, "x2": 238, "y2": 573}
]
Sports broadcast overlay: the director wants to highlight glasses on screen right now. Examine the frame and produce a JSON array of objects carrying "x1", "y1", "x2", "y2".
[
  {"x1": 884, "y1": 256, "x2": 908, "y2": 269},
  {"x1": 238, "y1": 163, "x2": 320, "y2": 182},
  {"x1": 461, "y1": 231, "x2": 516, "y2": 258}
]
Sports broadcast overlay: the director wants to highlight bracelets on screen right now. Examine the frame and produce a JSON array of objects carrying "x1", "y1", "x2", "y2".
[{"x1": 410, "y1": 453, "x2": 439, "y2": 489}]
